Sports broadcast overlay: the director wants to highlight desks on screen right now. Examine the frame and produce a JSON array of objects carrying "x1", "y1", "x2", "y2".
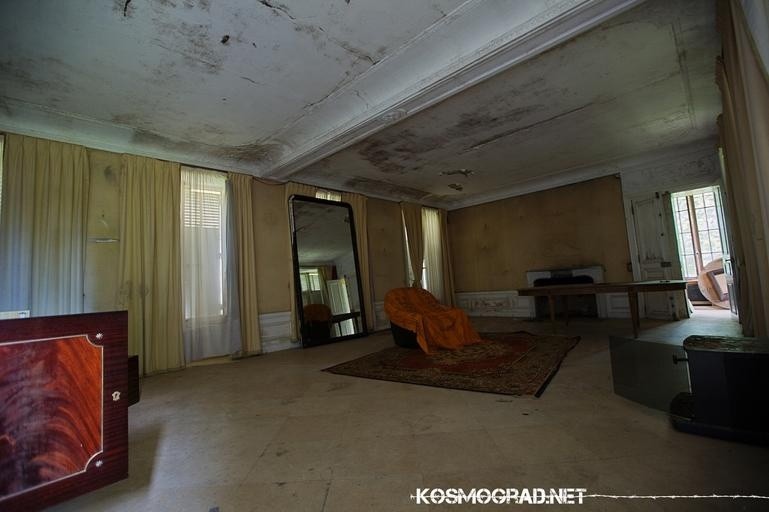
[
  {"x1": 333, "y1": 311, "x2": 361, "y2": 336},
  {"x1": 516, "y1": 279, "x2": 690, "y2": 338}
]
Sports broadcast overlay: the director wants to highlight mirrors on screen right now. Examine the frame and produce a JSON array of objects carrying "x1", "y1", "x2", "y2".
[{"x1": 287, "y1": 193, "x2": 368, "y2": 348}]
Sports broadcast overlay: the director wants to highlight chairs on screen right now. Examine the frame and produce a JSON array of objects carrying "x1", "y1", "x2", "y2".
[
  {"x1": 383, "y1": 286, "x2": 495, "y2": 355},
  {"x1": 304, "y1": 304, "x2": 333, "y2": 338}
]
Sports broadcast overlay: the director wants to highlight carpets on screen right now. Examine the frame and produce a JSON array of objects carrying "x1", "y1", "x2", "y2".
[{"x1": 319, "y1": 332, "x2": 581, "y2": 397}]
